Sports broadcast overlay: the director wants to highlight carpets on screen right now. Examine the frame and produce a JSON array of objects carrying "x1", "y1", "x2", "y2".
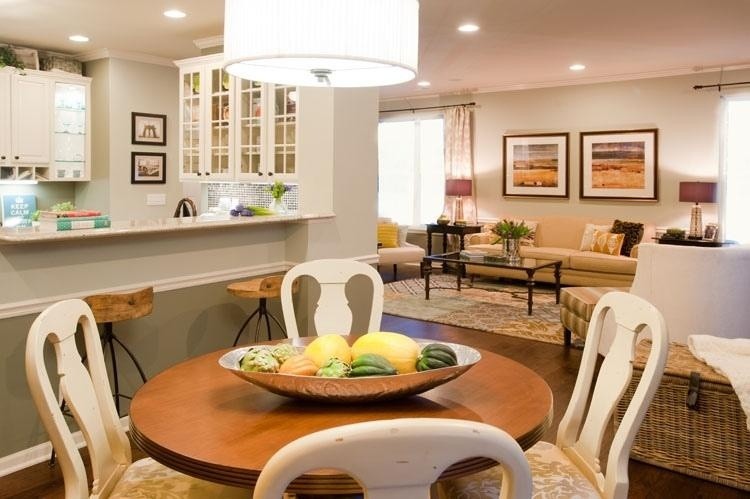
[{"x1": 383, "y1": 272, "x2": 585, "y2": 351}]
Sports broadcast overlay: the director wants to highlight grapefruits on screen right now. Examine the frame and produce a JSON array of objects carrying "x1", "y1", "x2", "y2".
[{"x1": 302, "y1": 334, "x2": 351, "y2": 368}]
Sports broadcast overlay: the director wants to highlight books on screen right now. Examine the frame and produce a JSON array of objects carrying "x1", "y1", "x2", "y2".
[
  {"x1": 30, "y1": 219, "x2": 112, "y2": 231},
  {"x1": 40, "y1": 208, "x2": 101, "y2": 217},
  {"x1": 37, "y1": 214, "x2": 110, "y2": 221}
]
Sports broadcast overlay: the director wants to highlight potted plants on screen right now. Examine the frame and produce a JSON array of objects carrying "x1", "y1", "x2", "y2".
[{"x1": 488, "y1": 218, "x2": 537, "y2": 262}]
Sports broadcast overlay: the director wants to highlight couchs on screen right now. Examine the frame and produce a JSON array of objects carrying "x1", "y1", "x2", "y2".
[
  {"x1": 377, "y1": 215, "x2": 426, "y2": 282},
  {"x1": 560, "y1": 287, "x2": 620, "y2": 348},
  {"x1": 462, "y1": 217, "x2": 642, "y2": 287}
]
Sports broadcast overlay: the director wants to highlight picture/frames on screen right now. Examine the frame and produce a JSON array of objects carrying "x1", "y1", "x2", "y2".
[
  {"x1": 502, "y1": 131, "x2": 570, "y2": 201},
  {"x1": 130, "y1": 151, "x2": 166, "y2": 185},
  {"x1": 579, "y1": 128, "x2": 659, "y2": 204},
  {"x1": 131, "y1": 111, "x2": 168, "y2": 146}
]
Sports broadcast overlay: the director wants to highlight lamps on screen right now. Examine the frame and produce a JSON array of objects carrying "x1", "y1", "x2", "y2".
[
  {"x1": 222, "y1": 0, "x2": 421, "y2": 92},
  {"x1": 445, "y1": 179, "x2": 473, "y2": 221},
  {"x1": 678, "y1": 181, "x2": 719, "y2": 240}
]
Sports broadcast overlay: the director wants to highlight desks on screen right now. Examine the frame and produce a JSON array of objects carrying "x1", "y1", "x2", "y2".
[
  {"x1": 650, "y1": 236, "x2": 738, "y2": 247},
  {"x1": 426, "y1": 221, "x2": 486, "y2": 269},
  {"x1": 126, "y1": 334, "x2": 557, "y2": 499}
]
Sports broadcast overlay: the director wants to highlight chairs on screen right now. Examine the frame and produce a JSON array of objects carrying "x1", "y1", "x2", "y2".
[
  {"x1": 22, "y1": 296, "x2": 257, "y2": 498},
  {"x1": 226, "y1": 274, "x2": 303, "y2": 347},
  {"x1": 250, "y1": 414, "x2": 536, "y2": 499},
  {"x1": 279, "y1": 258, "x2": 387, "y2": 338},
  {"x1": 436, "y1": 289, "x2": 670, "y2": 499},
  {"x1": 45, "y1": 284, "x2": 154, "y2": 470}
]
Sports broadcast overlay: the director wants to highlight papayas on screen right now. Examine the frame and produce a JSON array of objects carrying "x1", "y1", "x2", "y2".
[{"x1": 351, "y1": 331, "x2": 421, "y2": 374}]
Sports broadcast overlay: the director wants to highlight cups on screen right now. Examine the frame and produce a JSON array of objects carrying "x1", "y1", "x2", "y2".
[{"x1": 208, "y1": 194, "x2": 240, "y2": 212}]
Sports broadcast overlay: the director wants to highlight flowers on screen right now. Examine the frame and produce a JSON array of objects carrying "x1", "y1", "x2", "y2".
[
  {"x1": 261, "y1": 174, "x2": 292, "y2": 202},
  {"x1": 230, "y1": 202, "x2": 272, "y2": 217}
]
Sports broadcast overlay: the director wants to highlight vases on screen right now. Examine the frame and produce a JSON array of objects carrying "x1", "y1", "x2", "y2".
[{"x1": 267, "y1": 198, "x2": 289, "y2": 215}]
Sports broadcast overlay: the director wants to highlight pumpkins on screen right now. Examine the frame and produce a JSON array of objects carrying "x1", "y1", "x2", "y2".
[
  {"x1": 416, "y1": 343, "x2": 457, "y2": 372},
  {"x1": 349, "y1": 353, "x2": 399, "y2": 378},
  {"x1": 278, "y1": 355, "x2": 318, "y2": 376}
]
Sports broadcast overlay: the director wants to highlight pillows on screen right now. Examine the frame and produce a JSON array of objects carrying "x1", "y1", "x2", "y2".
[
  {"x1": 378, "y1": 221, "x2": 400, "y2": 247},
  {"x1": 576, "y1": 218, "x2": 645, "y2": 257},
  {"x1": 398, "y1": 223, "x2": 411, "y2": 247}
]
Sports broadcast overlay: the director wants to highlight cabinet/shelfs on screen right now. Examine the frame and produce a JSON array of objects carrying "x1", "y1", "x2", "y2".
[
  {"x1": 0, "y1": 64, "x2": 53, "y2": 183},
  {"x1": 173, "y1": 52, "x2": 235, "y2": 185},
  {"x1": 236, "y1": 75, "x2": 298, "y2": 186},
  {"x1": 49, "y1": 68, "x2": 94, "y2": 183}
]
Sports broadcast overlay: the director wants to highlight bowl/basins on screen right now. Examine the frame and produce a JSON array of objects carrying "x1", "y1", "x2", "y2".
[
  {"x1": 454, "y1": 220, "x2": 466, "y2": 227},
  {"x1": 218, "y1": 339, "x2": 482, "y2": 405},
  {"x1": 436, "y1": 219, "x2": 450, "y2": 224}
]
